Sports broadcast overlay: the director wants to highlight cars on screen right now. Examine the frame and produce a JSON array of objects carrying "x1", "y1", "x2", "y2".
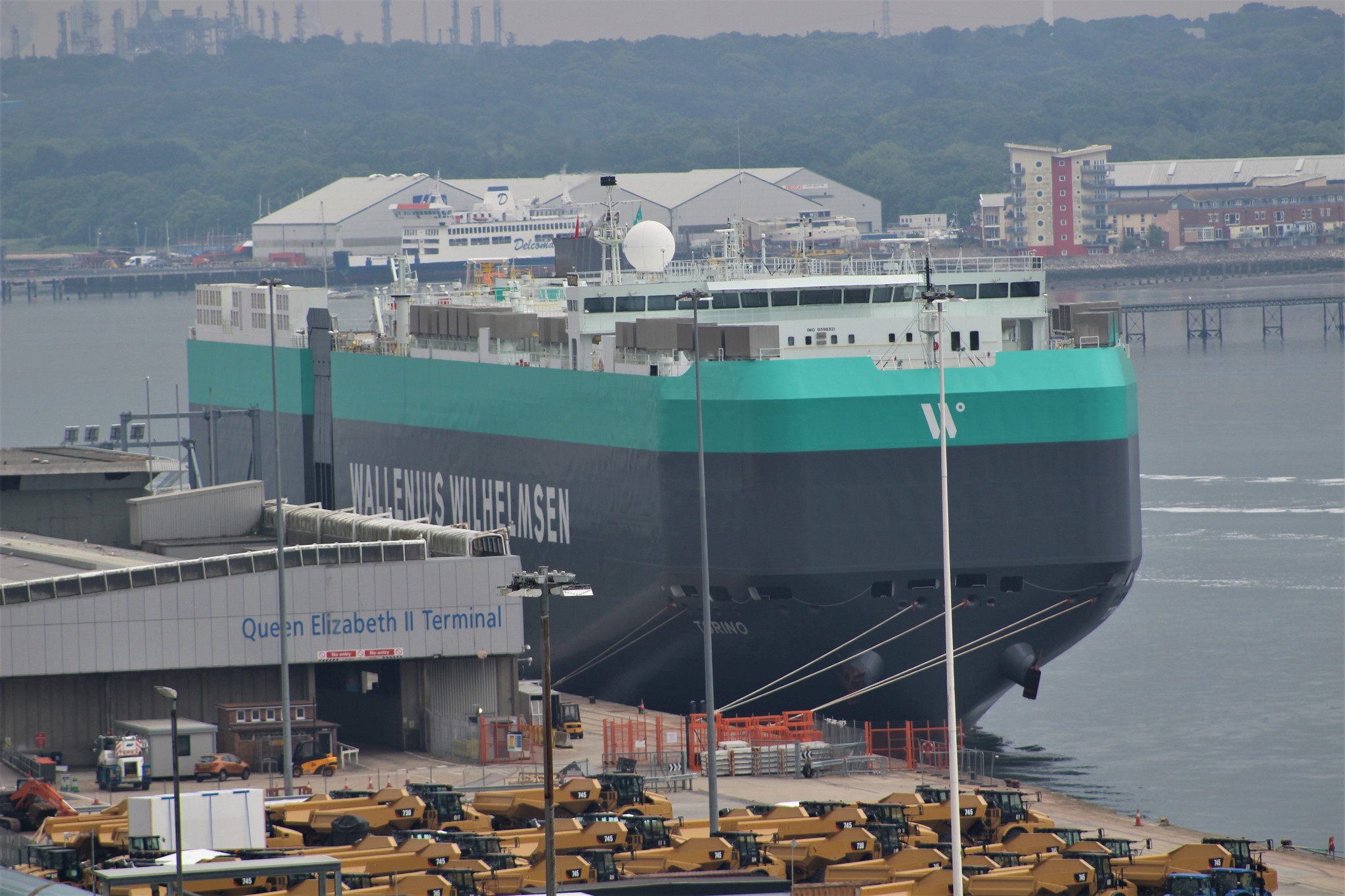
[
  {"x1": 193, "y1": 752, "x2": 251, "y2": 783},
  {"x1": 556, "y1": 700, "x2": 584, "y2": 739},
  {"x1": 292, "y1": 739, "x2": 338, "y2": 778}
]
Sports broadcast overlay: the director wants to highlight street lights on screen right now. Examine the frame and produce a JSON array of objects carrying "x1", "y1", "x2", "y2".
[
  {"x1": 921, "y1": 289, "x2": 964, "y2": 896},
  {"x1": 152, "y1": 684, "x2": 184, "y2": 896},
  {"x1": 254, "y1": 278, "x2": 296, "y2": 798},
  {"x1": 498, "y1": 565, "x2": 594, "y2": 896},
  {"x1": 673, "y1": 288, "x2": 723, "y2": 837}
]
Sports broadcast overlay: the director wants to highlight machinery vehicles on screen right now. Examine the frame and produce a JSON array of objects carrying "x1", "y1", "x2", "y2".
[{"x1": 0, "y1": 775, "x2": 80, "y2": 834}]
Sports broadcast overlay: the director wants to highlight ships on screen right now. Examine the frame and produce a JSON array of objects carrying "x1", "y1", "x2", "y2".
[{"x1": 180, "y1": 196, "x2": 1149, "y2": 751}]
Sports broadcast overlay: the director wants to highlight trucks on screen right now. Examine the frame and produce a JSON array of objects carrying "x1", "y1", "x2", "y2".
[{"x1": 91, "y1": 731, "x2": 153, "y2": 793}]
